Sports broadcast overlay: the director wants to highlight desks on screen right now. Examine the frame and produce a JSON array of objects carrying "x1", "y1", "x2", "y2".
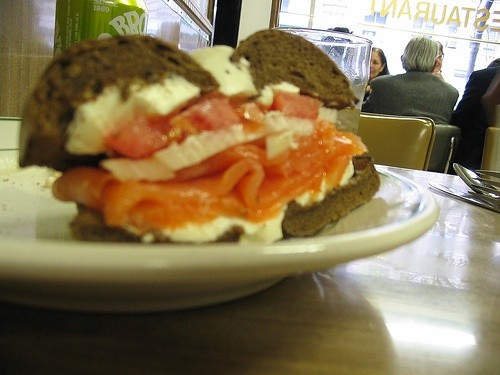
[{"x1": 0, "y1": 164, "x2": 500, "y2": 375}]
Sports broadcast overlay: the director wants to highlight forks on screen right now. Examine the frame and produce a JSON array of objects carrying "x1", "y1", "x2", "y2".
[{"x1": 468, "y1": 170, "x2": 500, "y2": 209}]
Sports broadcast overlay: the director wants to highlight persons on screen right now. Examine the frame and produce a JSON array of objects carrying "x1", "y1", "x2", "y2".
[
  {"x1": 452, "y1": 59, "x2": 500, "y2": 176},
  {"x1": 320, "y1": 27, "x2": 390, "y2": 110},
  {"x1": 361, "y1": 37, "x2": 459, "y2": 126}
]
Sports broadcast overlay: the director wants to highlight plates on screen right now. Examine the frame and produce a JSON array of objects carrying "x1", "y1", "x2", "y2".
[{"x1": 0, "y1": 167, "x2": 439, "y2": 313}]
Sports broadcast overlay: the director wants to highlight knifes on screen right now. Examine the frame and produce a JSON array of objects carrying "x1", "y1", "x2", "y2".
[{"x1": 428, "y1": 181, "x2": 500, "y2": 212}]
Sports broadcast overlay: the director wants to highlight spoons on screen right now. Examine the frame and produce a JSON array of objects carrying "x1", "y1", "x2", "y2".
[{"x1": 453, "y1": 163, "x2": 500, "y2": 198}]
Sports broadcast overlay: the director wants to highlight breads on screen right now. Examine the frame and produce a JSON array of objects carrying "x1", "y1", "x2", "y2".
[{"x1": 20, "y1": 32, "x2": 380, "y2": 244}]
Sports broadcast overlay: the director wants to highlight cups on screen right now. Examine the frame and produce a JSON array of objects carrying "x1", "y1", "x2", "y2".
[{"x1": 272, "y1": 27, "x2": 373, "y2": 137}]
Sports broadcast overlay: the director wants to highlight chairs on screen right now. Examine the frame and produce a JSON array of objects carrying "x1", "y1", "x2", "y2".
[{"x1": 358, "y1": 113, "x2": 500, "y2": 173}]
